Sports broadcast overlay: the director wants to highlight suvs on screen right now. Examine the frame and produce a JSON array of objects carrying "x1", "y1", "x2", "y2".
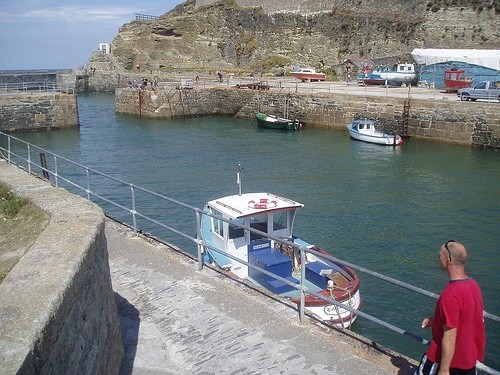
[{"x1": 176, "y1": 79, "x2": 195, "y2": 89}]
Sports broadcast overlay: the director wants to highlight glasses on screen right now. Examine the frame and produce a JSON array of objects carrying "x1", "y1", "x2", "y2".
[{"x1": 444, "y1": 239, "x2": 455, "y2": 262}]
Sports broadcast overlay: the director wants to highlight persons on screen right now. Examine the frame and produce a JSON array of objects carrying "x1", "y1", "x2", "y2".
[
  {"x1": 127, "y1": 76, "x2": 199, "y2": 89},
  {"x1": 413, "y1": 240, "x2": 486, "y2": 375}
]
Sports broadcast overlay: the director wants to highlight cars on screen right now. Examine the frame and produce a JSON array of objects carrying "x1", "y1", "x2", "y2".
[{"x1": 457, "y1": 81, "x2": 500, "y2": 102}]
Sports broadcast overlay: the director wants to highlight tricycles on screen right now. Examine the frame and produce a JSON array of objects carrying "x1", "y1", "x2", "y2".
[
  {"x1": 443, "y1": 69, "x2": 473, "y2": 89},
  {"x1": 274, "y1": 69, "x2": 285, "y2": 78}
]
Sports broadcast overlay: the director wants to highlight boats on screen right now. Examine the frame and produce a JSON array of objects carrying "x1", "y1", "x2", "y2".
[
  {"x1": 345, "y1": 117, "x2": 402, "y2": 146},
  {"x1": 290, "y1": 67, "x2": 327, "y2": 82},
  {"x1": 254, "y1": 98, "x2": 302, "y2": 130},
  {"x1": 199, "y1": 161, "x2": 363, "y2": 330},
  {"x1": 359, "y1": 64, "x2": 417, "y2": 86}
]
